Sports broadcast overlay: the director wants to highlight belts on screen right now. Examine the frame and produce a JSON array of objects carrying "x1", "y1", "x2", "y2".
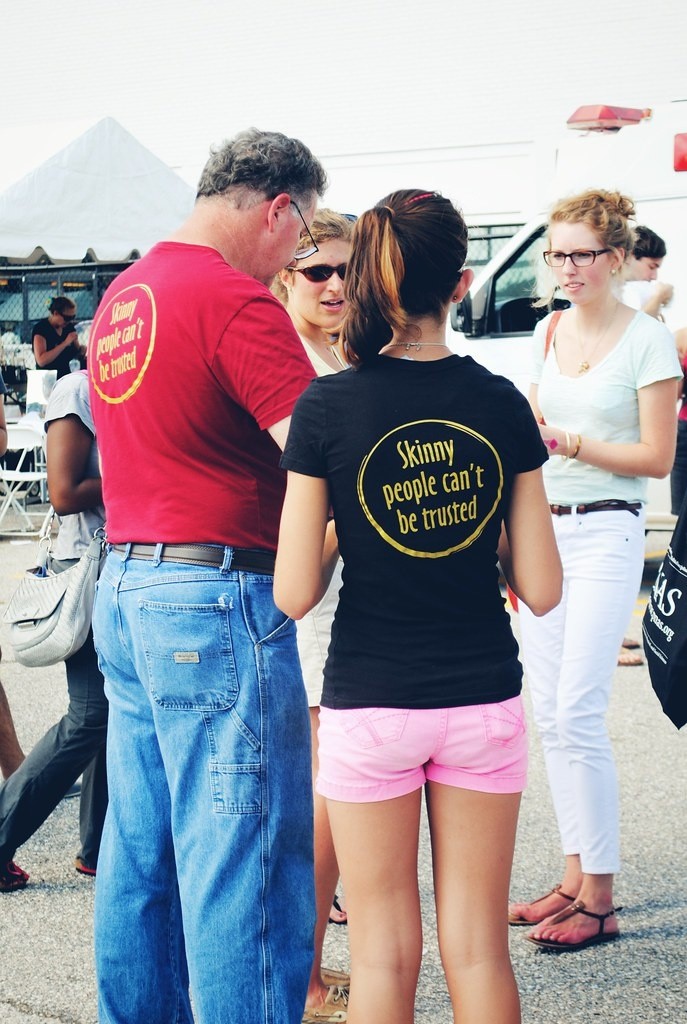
[
  {"x1": 112, "y1": 544, "x2": 275, "y2": 575},
  {"x1": 549, "y1": 499, "x2": 643, "y2": 518}
]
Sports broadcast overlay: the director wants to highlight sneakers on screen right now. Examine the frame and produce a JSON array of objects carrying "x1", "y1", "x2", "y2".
[
  {"x1": 300, "y1": 984, "x2": 351, "y2": 1024},
  {"x1": 321, "y1": 966, "x2": 351, "y2": 987}
]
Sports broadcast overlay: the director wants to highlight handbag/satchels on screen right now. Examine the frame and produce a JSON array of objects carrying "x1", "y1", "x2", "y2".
[{"x1": 3, "y1": 525, "x2": 109, "y2": 667}]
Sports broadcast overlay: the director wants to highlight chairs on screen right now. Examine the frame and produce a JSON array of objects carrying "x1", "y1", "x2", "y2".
[{"x1": 0, "y1": 423, "x2": 53, "y2": 541}]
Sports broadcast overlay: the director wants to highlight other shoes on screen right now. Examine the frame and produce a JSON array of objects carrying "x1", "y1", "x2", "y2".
[
  {"x1": 0, "y1": 861, "x2": 30, "y2": 893},
  {"x1": 64, "y1": 781, "x2": 84, "y2": 797},
  {"x1": 76, "y1": 858, "x2": 95, "y2": 875}
]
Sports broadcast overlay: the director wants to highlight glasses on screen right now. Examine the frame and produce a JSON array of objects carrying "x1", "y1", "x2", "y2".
[
  {"x1": 543, "y1": 249, "x2": 612, "y2": 268},
  {"x1": 58, "y1": 312, "x2": 75, "y2": 322},
  {"x1": 282, "y1": 262, "x2": 347, "y2": 283},
  {"x1": 267, "y1": 197, "x2": 319, "y2": 260}
]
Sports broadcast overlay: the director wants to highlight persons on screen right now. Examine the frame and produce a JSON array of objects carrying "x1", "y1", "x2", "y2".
[
  {"x1": 270, "y1": 190, "x2": 564, "y2": 1024},
  {"x1": 0, "y1": 365, "x2": 125, "y2": 892},
  {"x1": 613, "y1": 225, "x2": 672, "y2": 667},
  {"x1": 33, "y1": 296, "x2": 89, "y2": 377},
  {"x1": 85, "y1": 128, "x2": 328, "y2": 1024},
  {"x1": 508, "y1": 192, "x2": 683, "y2": 949}
]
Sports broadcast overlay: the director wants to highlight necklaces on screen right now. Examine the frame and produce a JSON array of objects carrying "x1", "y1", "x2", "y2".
[
  {"x1": 572, "y1": 301, "x2": 619, "y2": 371},
  {"x1": 381, "y1": 341, "x2": 447, "y2": 350}
]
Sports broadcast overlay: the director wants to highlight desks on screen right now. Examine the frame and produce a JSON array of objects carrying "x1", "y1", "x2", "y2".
[{"x1": 5, "y1": 418, "x2": 47, "y2": 504}]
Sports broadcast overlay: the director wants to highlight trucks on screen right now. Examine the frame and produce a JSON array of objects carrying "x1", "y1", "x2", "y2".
[{"x1": 444, "y1": 104, "x2": 687, "y2": 532}]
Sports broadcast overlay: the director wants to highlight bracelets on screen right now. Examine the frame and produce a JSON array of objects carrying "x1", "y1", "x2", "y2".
[
  {"x1": 570, "y1": 432, "x2": 581, "y2": 460},
  {"x1": 560, "y1": 430, "x2": 569, "y2": 463}
]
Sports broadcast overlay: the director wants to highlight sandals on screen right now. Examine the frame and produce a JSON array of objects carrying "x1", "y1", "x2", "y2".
[
  {"x1": 328, "y1": 895, "x2": 349, "y2": 924},
  {"x1": 508, "y1": 885, "x2": 579, "y2": 926},
  {"x1": 523, "y1": 902, "x2": 622, "y2": 951}
]
